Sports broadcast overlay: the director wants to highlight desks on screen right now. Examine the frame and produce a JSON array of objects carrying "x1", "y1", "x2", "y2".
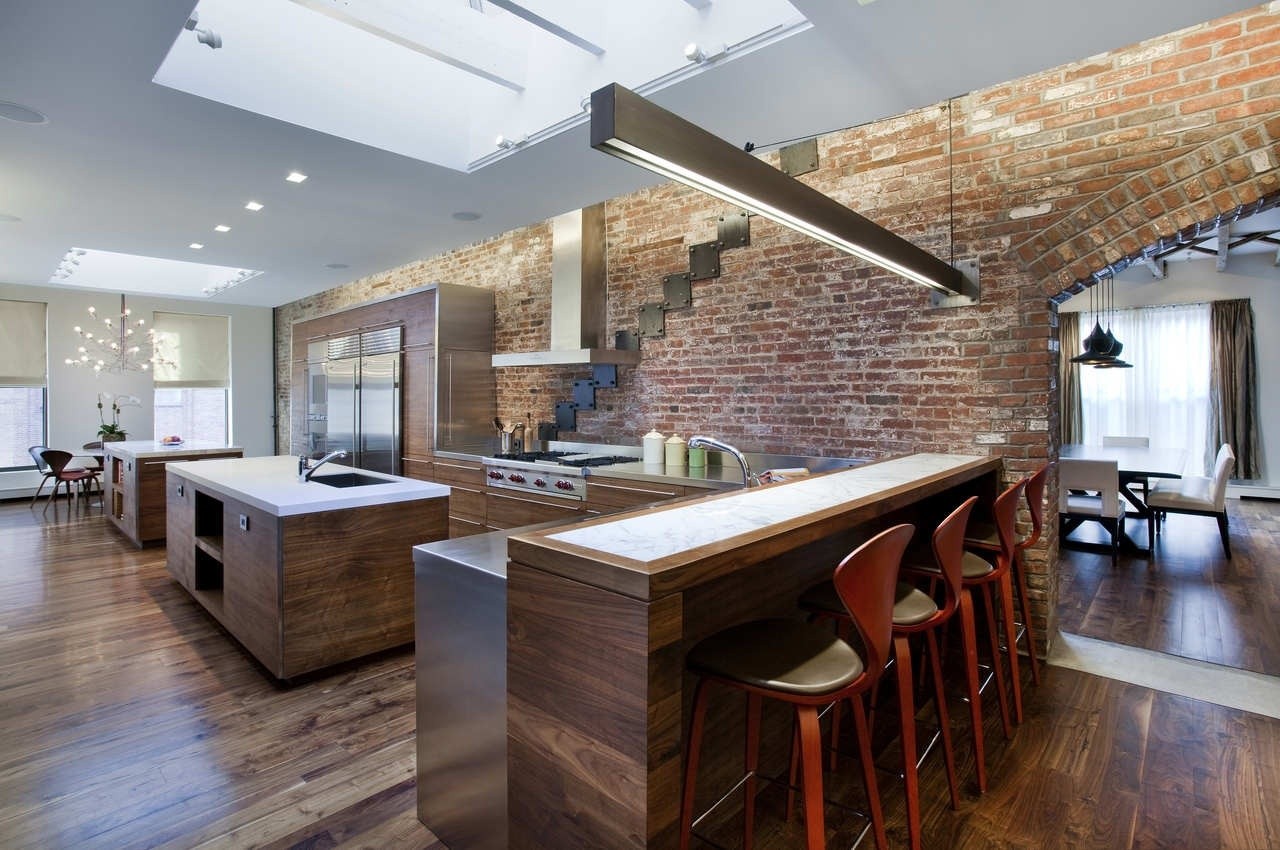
[
  {"x1": 1058, "y1": 442, "x2": 1185, "y2": 550},
  {"x1": 61, "y1": 448, "x2": 104, "y2": 457},
  {"x1": 503, "y1": 453, "x2": 1003, "y2": 850}
]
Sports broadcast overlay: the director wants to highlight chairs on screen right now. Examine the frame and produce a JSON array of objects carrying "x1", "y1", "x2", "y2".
[
  {"x1": 676, "y1": 521, "x2": 916, "y2": 850},
  {"x1": 784, "y1": 491, "x2": 981, "y2": 850},
  {"x1": 1094, "y1": 437, "x2": 1151, "y2": 521},
  {"x1": 1146, "y1": 442, "x2": 1236, "y2": 562},
  {"x1": 29, "y1": 445, "x2": 85, "y2": 508},
  {"x1": 1059, "y1": 456, "x2": 1126, "y2": 566},
  {"x1": 40, "y1": 450, "x2": 103, "y2": 515},
  {"x1": 925, "y1": 460, "x2": 1051, "y2": 728},
  {"x1": 861, "y1": 478, "x2": 1030, "y2": 805},
  {"x1": 83, "y1": 441, "x2": 105, "y2": 505}
]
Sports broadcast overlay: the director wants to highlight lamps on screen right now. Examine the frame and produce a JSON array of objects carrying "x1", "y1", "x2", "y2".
[
  {"x1": 187, "y1": 10, "x2": 222, "y2": 54},
  {"x1": 685, "y1": 43, "x2": 727, "y2": 66},
  {"x1": 1068, "y1": 275, "x2": 1134, "y2": 369},
  {"x1": 588, "y1": 82, "x2": 969, "y2": 298},
  {"x1": 494, "y1": 132, "x2": 532, "y2": 147},
  {"x1": 65, "y1": 293, "x2": 180, "y2": 376}
]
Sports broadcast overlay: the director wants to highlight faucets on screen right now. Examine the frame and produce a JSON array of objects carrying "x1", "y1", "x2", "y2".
[
  {"x1": 301, "y1": 448, "x2": 349, "y2": 482},
  {"x1": 687, "y1": 435, "x2": 755, "y2": 489}
]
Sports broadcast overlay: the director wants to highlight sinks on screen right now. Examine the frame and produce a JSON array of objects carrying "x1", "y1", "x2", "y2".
[{"x1": 307, "y1": 472, "x2": 396, "y2": 489}]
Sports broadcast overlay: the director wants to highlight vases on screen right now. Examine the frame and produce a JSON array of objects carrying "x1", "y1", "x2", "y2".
[{"x1": 100, "y1": 434, "x2": 127, "y2": 451}]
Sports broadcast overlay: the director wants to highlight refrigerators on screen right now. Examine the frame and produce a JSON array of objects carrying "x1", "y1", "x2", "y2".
[{"x1": 326, "y1": 324, "x2": 402, "y2": 476}]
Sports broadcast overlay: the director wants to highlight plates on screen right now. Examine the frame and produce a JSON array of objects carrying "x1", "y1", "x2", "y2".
[{"x1": 160, "y1": 440, "x2": 185, "y2": 446}]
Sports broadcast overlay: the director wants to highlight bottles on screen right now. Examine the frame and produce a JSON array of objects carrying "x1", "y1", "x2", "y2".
[{"x1": 523, "y1": 413, "x2": 534, "y2": 453}]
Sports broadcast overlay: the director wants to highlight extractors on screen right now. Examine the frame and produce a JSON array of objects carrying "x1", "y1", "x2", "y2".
[{"x1": 490, "y1": 207, "x2": 637, "y2": 367}]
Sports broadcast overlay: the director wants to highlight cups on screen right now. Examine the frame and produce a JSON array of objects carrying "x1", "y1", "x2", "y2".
[
  {"x1": 663, "y1": 433, "x2": 686, "y2": 467},
  {"x1": 500, "y1": 431, "x2": 515, "y2": 453},
  {"x1": 689, "y1": 448, "x2": 708, "y2": 469},
  {"x1": 641, "y1": 429, "x2": 665, "y2": 465}
]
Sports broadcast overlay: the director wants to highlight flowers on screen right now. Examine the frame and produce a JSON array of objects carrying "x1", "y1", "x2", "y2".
[{"x1": 97, "y1": 392, "x2": 143, "y2": 438}]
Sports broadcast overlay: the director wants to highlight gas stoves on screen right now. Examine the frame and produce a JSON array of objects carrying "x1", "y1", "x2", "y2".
[{"x1": 483, "y1": 449, "x2": 642, "y2": 502}]
